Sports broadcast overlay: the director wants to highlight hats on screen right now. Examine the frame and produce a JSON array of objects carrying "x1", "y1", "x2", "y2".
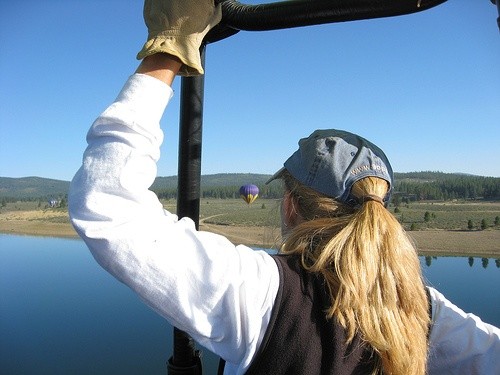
[{"x1": 265, "y1": 128, "x2": 394, "y2": 209}]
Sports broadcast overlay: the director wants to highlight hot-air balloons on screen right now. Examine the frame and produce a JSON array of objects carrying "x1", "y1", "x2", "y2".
[{"x1": 238, "y1": 184, "x2": 260, "y2": 208}]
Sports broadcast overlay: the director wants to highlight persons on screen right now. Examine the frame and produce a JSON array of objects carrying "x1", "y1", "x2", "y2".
[{"x1": 68, "y1": 2, "x2": 500, "y2": 374}]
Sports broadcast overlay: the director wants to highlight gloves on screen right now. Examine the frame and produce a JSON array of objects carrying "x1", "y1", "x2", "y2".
[{"x1": 134, "y1": 0, "x2": 223, "y2": 77}]
